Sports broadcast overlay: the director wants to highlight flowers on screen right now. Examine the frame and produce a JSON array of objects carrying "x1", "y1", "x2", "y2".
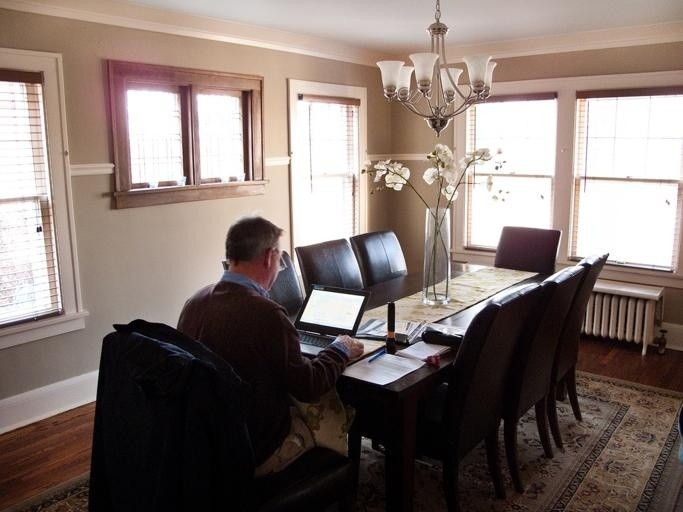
[{"x1": 361, "y1": 146, "x2": 492, "y2": 300}]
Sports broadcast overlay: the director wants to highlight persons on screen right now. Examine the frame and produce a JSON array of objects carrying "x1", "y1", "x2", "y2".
[{"x1": 175, "y1": 218, "x2": 366, "y2": 479}]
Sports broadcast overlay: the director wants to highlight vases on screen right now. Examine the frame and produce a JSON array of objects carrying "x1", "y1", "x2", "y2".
[{"x1": 422, "y1": 208, "x2": 452, "y2": 304}]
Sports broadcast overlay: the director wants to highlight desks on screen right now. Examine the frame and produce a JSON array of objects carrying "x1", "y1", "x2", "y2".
[{"x1": 283, "y1": 261, "x2": 549, "y2": 512}]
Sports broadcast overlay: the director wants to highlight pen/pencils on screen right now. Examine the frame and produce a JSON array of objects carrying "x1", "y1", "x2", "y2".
[{"x1": 367, "y1": 349, "x2": 386, "y2": 363}]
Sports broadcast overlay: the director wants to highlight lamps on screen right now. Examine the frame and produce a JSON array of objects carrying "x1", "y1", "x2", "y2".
[{"x1": 376, "y1": 0, "x2": 496, "y2": 134}]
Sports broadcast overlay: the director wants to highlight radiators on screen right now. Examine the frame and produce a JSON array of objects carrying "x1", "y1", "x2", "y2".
[{"x1": 580, "y1": 278, "x2": 665, "y2": 356}]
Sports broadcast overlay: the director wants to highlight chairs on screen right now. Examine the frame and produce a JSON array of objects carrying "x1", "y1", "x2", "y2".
[
  {"x1": 295, "y1": 239, "x2": 364, "y2": 305},
  {"x1": 89, "y1": 321, "x2": 351, "y2": 512},
  {"x1": 547, "y1": 257, "x2": 607, "y2": 448},
  {"x1": 372, "y1": 288, "x2": 542, "y2": 511},
  {"x1": 506, "y1": 266, "x2": 586, "y2": 495},
  {"x1": 222, "y1": 251, "x2": 303, "y2": 316},
  {"x1": 350, "y1": 230, "x2": 408, "y2": 287},
  {"x1": 494, "y1": 226, "x2": 561, "y2": 274}
]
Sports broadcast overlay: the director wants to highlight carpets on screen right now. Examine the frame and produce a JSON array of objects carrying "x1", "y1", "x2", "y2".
[{"x1": 0, "y1": 370, "x2": 683, "y2": 512}]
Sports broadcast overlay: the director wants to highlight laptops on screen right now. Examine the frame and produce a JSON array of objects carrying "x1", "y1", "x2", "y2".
[{"x1": 293, "y1": 283, "x2": 372, "y2": 359}]
